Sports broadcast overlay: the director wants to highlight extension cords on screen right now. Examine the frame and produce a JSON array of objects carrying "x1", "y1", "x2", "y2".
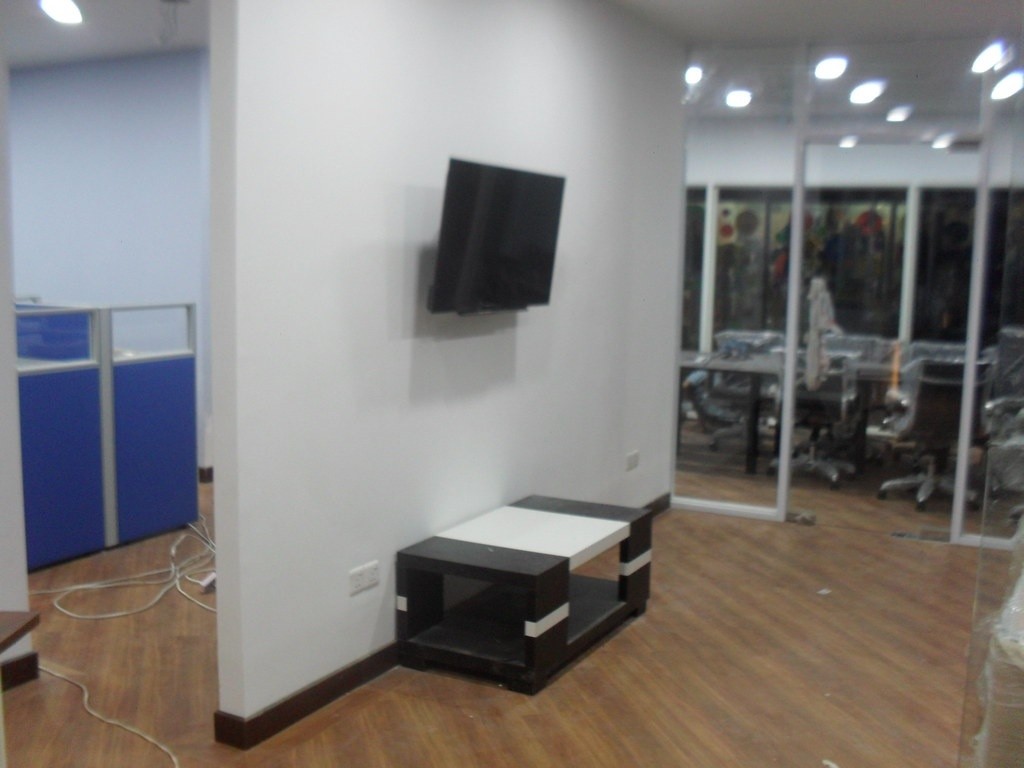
[{"x1": 200, "y1": 572, "x2": 216, "y2": 593}]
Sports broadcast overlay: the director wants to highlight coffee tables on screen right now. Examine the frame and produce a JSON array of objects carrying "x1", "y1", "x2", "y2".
[{"x1": 394, "y1": 496, "x2": 651, "y2": 694}]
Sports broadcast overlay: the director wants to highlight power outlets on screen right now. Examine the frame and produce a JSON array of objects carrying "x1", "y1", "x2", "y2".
[
  {"x1": 625, "y1": 450, "x2": 639, "y2": 472},
  {"x1": 347, "y1": 561, "x2": 380, "y2": 594}
]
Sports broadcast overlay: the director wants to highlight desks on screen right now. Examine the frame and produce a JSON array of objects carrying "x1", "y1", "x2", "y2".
[{"x1": 680, "y1": 350, "x2": 782, "y2": 476}]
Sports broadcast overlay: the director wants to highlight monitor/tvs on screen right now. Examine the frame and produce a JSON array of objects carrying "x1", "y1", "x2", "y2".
[{"x1": 422, "y1": 157, "x2": 565, "y2": 314}]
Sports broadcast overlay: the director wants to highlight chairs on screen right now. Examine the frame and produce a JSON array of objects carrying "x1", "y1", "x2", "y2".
[
  {"x1": 876, "y1": 363, "x2": 988, "y2": 511},
  {"x1": 767, "y1": 394, "x2": 855, "y2": 488},
  {"x1": 842, "y1": 369, "x2": 903, "y2": 468}
]
